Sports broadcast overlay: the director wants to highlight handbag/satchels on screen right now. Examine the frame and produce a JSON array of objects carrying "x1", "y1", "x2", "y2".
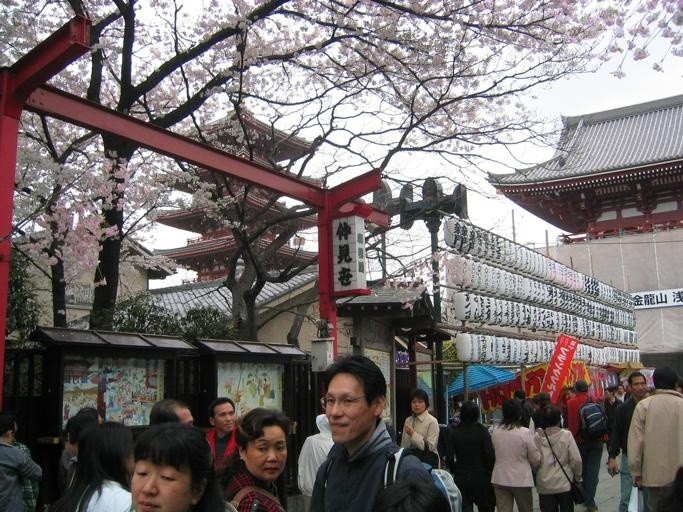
[
  {"x1": 410, "y1": 440, "x2": 439, "y2": 470},
  {"x1": 544, "y1": 429, "x2": 585, "y2": 504}
]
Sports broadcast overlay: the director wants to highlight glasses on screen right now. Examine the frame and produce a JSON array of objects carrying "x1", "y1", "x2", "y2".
[{"x1": 320, "y1": 395, "x2": 371, "y2": 409}]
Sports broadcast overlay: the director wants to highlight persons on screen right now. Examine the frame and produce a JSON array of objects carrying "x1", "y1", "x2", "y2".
[
  {"x1": 608, "y1": 371, "x2": 651, "y2": 511},
  {"x1": 603, "y1": 389, "x2": 622, "y2": 423},
  {"x1": 1, "y1": 418, "x2": 41, "y2": 512},
  {"x1": 13, "y1": 439, "x2": 41, "y2": 511},
  {"x1": 561, "y1": 389, "x2": 574, "y2": 402},
  {"x1": 299, "y1": 412, "x2": 334, "y2": 510},
  {"x1": 400, "y1": 389, "x2": 440, "y2": 471},
  {"x1": 533, "y1": 407, "x2": 583, "y2": 512},
  {"x1": 44, "y1": 407, "x2": 137, "y2": 512},
  {"x1": 150, "y1": 400, "x2": 193, "y2": 425},
  {"x1": 514, "y1": 387, "x2": 534, "y2": 420},
  {"x1": 627, "y1": 366, "x2": 683, "y2": 511},
  {"x1": 444, "y1": 402, "x2": 494, "y2": 512},
  {"x1": 615, "y1": 384, "x2": 626, "y2": 400},
  {"x1": 222, "y1": 408, "x2": 287, "y2": 512},
  {"x1": 565, "y1": 380, "x2": 601, "y2": 511},
  {"x1": 531, "y1": 393, "x2": 550, "y2": 412},
  {"x1": 308, "y1": 356, "x2": 454, "y2": 512},
  {"x1": 127, "y1": 423, "x2": 214, "y2": 511},
  {"x1": 205, "y1": 398, "x2": 241, "y2": 471},
  {"x1": 489, "y1": 400, "x2": 542, "y2": 511}
]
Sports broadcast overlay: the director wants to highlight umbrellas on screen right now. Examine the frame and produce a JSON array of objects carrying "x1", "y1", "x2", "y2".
[{"x1": 444, "y1": 363, "x2": 516, "y2": 398}]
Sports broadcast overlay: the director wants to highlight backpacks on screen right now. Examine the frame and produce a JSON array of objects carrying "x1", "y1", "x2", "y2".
[
  {"x1": 324, "y1": 448, "x2": 462, "y2": 512},
  {"x1": 578, "y1": 395, "x2": 609, "y2": 443}
]
